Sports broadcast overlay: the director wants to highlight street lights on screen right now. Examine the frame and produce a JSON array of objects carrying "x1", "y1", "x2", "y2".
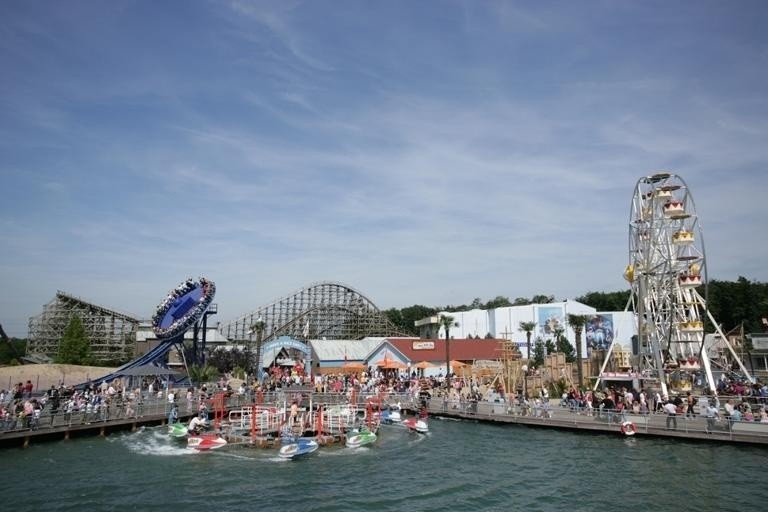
[
  {"x1": 406, "y1": 363, "x2": 414, "y2": 388},
  {"x1": 521, "y1": 365, "x2": 529, "y2": 402}
]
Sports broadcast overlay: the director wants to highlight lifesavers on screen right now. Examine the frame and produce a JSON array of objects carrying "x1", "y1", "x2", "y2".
[{"x1": 622, "y1": 421, "x2": 637, "y2": 436}]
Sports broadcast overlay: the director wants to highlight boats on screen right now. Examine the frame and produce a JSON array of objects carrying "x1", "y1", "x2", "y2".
[{"x1": 165, "y1": 404, "x2": 432, "y2": 464}]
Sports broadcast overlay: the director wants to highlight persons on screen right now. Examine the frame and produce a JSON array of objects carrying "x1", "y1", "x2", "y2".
[
  {"x1": 0, "y1": 358, "x2": 767, "y2": 433},
  {"x1": 151, "y1": 274, "x2": 214, "y2": 337}
]
